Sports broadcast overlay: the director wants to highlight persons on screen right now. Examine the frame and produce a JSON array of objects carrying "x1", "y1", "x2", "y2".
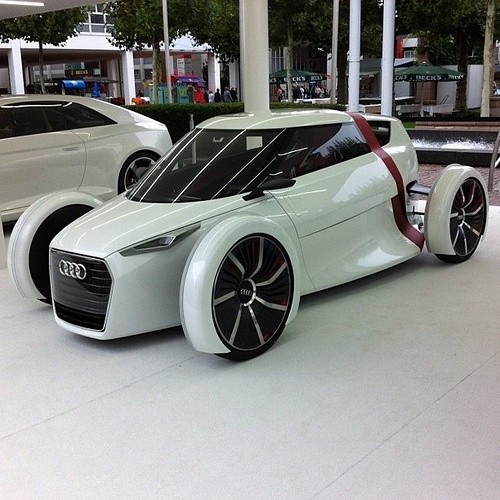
[
  {"x1": 195, "y1": 84, "x2": 328, "y2": 104},
  {"x1": 136, "y1": 90, "x2": 144, "y2": 97}
]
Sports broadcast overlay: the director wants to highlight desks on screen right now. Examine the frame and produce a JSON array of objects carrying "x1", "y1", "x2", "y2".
[
  {"x1": 359, "y1": 96, "x2": 415, "y2": 118},
  {"x1": 302, "y1": 98, "x2": 338, "y2": 107}
]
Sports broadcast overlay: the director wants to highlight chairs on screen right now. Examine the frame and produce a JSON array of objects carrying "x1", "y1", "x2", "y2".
[
  {"x1": 423, "y1": 104, "x2": 452, "y2": 116},
  {"x1": 397, "y1": 104, "x2": 422, "y2": 117},
  {"x1": 360, "y1": 105, "x2": 381, "y2": 114}
]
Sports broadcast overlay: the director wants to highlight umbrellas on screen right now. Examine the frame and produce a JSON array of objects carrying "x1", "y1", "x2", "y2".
[
  {"x1": 269, "y1": 69, "x2": 327, "y2": 85},
  {"x1": 393, "y1": 61, "x2": 466, "y2": 106}
]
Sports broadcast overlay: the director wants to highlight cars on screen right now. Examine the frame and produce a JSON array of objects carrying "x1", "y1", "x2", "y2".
[
  {"x1": 6, "y1": 108, "x2": 490, "y2": 363},
  {"x1": 0, "y1": 94, "x2": 173, "y2": 228}
]
